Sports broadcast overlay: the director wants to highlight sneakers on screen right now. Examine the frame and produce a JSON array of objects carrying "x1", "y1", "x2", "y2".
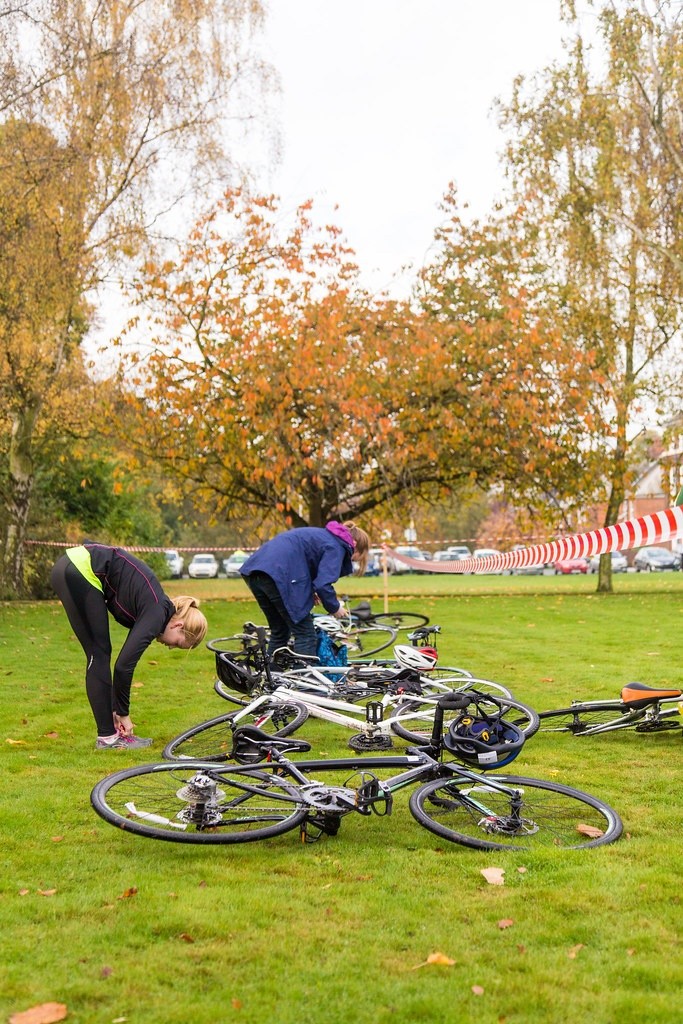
[{"x1": 97, "y1": 732, "x2": 154, "y2": 749}]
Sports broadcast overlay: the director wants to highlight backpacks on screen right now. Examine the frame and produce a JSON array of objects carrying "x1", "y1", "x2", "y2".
[{"x1": 314, "y1": 627, "x2": 349, "y2": 685}]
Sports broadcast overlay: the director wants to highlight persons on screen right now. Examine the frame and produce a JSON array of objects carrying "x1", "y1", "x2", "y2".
[
  {"x1": 50, "y1": 542, "x2": 208, "y2": 748},
  {"x1": 241, "y1": 521, "x2": 370, "y2": 667}
]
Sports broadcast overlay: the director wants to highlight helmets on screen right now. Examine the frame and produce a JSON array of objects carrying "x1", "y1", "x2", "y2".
[
  {"x1": 313, "y1": 616, "x2": 345, "y2": 638},
  {"x1": 394, "y1": 644, "x2": 438, "y2": 672},
  {"x1": 442, "y1": 713, "x2": 525, "y2": 768}
]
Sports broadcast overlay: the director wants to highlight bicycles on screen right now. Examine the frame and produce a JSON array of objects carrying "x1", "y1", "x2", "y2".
[
  {"x1": 91, "y1": 603, "x2": 541, "y2": 762},
  {"x1": 90, "y1": 692, "x2": 624, "y2": 853},
  {"x1": 511, "y1": 683, "x2": 683, "y2": 737}
]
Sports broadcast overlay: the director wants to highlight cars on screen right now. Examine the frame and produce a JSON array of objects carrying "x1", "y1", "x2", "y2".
[
  {"x1": 589, "y1": 552, "x2": 628, "y2": 574},
  {"x1": 632, "y1": 547, "x2": 681, "y2": 573},
  {"x1": 352, "y1": 546, "x2": 544, "y2": 576},
  {"x1": 554, "y1": 558, "x2": 589, "y2": 575},
  {"x1": 164, "y1": 551, "x2": 184, "y2": 579},
  {"x1": 187, "y1": 554, "x2": 219, "y2": 579},
  {"x1": 223, "y1": 555, "x2": 250, "y2": 579}
]
